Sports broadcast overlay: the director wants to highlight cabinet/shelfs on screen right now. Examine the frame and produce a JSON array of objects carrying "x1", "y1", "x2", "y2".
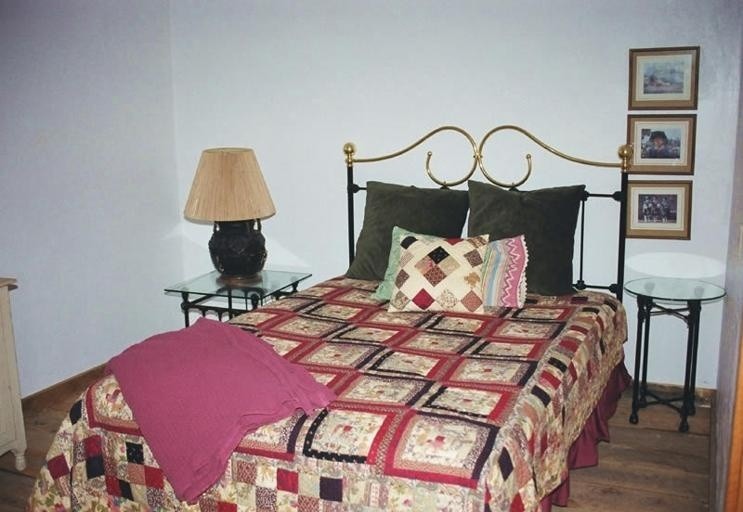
[{"x1": 1, "y1": 278, "x2": 28, "y2": 474}]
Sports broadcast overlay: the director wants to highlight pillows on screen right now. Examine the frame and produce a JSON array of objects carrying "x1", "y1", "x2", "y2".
[
  {"x1": 345, "y1": 180, "x2": 468, "y2": 281},
  {"x1": 468, "y1": 180, "x2": 586, "y2": 297},
  {"x1": 387, "y1": 232, "x2": 490, "y2": 316},
  {"x1": 481, "y1": 234, "x2": 529, "y2": 309},
  {"x1": 371, "y1": 226, "x2": 441, "y2": 301}
]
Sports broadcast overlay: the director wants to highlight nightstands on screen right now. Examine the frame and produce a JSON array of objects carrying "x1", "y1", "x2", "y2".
[{"x1": 164, "y1": 269, "x2": 313, "y2": 330}]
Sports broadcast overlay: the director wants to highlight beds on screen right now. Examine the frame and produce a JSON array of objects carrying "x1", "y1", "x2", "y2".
[{"x1": 25, "y1": 126, "x2": 632, "y2": 511}]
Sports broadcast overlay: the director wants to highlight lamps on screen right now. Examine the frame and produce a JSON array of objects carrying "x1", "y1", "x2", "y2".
[{"x1": 184, "y1": 148, "x2": 276, "y2": 285}]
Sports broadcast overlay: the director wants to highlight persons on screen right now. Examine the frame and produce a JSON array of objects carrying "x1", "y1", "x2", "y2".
[
  {"x1": 646, "y1": 131, "x2": 671, "y2": 158},
  {"x1": 643, "y1": 196, "x2": 670, "y2": 225}
]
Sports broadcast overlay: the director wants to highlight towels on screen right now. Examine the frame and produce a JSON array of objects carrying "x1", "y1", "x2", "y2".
[{"x1": 106, "y1": 316, "x2": 339, "y2": 503}]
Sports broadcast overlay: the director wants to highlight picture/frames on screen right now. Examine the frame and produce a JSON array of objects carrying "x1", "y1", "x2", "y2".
[
  {"x1": 625, "y1": 180, "x2": 693, "y2": 240},
  {"x1": 627, "y1": 114, "x2": 697, "y2": 176},
  {"x1": 628, "y1": 46, "x2": 700, "y2": 111}
]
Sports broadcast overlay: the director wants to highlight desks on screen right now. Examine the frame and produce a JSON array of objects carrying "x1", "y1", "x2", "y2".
[{"x1": 623, "y1": 276, "x2": 727, "y2": 432}]
